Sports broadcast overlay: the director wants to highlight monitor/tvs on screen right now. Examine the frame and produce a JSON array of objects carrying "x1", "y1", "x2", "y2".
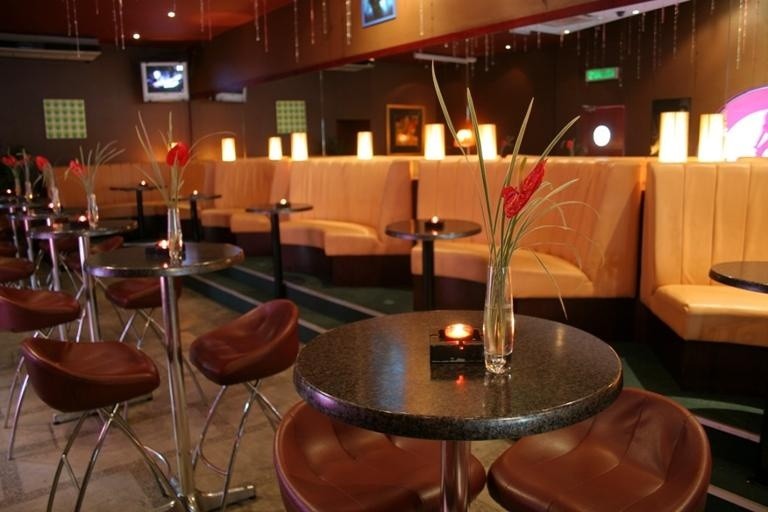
[
  {"x1": 141, "y1": 60, "x2": 191, "y2": 104},
  {"x1": 214, "y1": 85, "x2": 248, "y2": 104}
]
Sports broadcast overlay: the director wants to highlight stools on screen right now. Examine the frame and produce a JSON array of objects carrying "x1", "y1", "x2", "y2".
[
  {"x1": 188, "y1": 297, "x2": 300, "y2": 510},
  {"x1": 0, "y1": 240, "x2": 18, "y2": 257},
  {"x1": 40, "y1": 237, "x2": 80, "y2": 293},
  {"x1": 22, "y1": 337, "x2": 173, "y2": 512},
  {"x1": 0, "y1": 283, "x2": 83, "y2": 459},
  {"x1": 488, "y1": 387, "x2": 713, "y2": 510},
  {"x1": 273, "y1": 396, "x2": 485, "y2": 512},
  {"x1": 0, "y1": 255, "x2": 34, "y2": 288},
  {"x1": 104, "y1": 274, "x2": 209, "y2": 407},
  {"x1": 65, "y1": 235, "x2": 128, "y2": 338}
]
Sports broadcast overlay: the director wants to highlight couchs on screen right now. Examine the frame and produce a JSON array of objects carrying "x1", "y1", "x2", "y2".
[
  {"x1": 411, "y1": 154, "x2": 642, "y2": 328},
  {"x1": 638, "y1": 154, "x2": 767, "y2": 368},
  {"x1": 280, "y1": 157, "x2": 415, "y2": 292},
  {"x1": 201, "y1": 159, "x2": 287, "y2": 256},
  {"x1": 50, "y1": 160, "x2": 215, "y2": 224}
]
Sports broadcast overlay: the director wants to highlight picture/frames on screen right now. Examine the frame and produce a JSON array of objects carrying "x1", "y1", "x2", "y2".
[
  {"x1": 360, "y1": 0, "x2": 398, "y2": 28},
  {"x1": 385, "y1": 103, "x2": 425, "y2": 155}
]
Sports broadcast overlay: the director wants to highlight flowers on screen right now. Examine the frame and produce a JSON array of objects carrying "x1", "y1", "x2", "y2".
[
  {"x1": 33, "y1": 155, "x2": 55, "y2": 189},
  {"x1": 0, "y1": 154, "x2": 21, "y2": 177},
  {"x1": 434, "y1": 70, "x2": 601, "y2": 321},
  {"x1": 17, "y1": 148, "x2": 32, "y2": 182},
  {"x1": 64, "y1": 140, "x2": 125, "y2": 192},
  {"x1": 131, "y1": 111, "x2": 238, "y2": 207}
]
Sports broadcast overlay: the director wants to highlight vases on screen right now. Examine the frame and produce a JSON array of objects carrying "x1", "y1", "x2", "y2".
[
  {"x1": 48, "y1": 188, "x2": 63, "y2": 218},
  {"x1": 167, "y1": 208, "x2": 184, "y2": 265},
  {"x1": 87, "y1": 194, "x2": 99, "y2": 230},
  {"x1": 25, "y1": 181, "x2": 33, "y2": 205},
  {"x1": 15, "y1": 177, "x2": 22, "y2": 198},
  {"x1": 483, "y1": 263, "x2": 514, "y2": 374}
]
